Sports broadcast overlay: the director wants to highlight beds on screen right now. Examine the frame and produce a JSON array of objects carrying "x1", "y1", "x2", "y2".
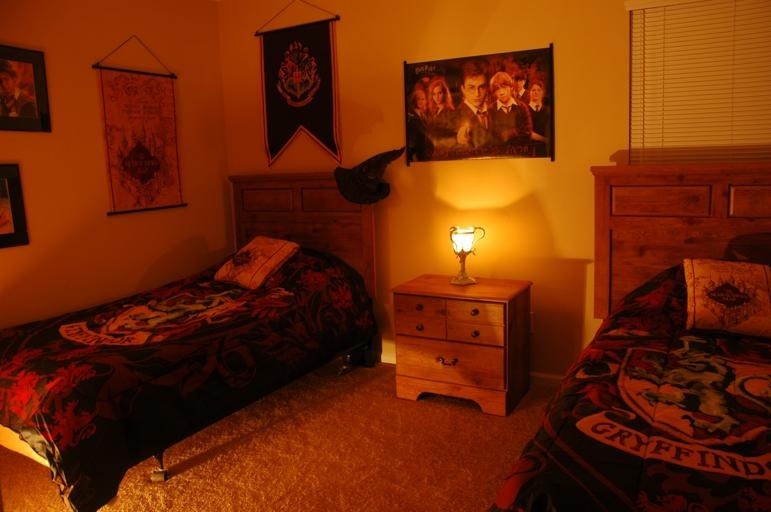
[
  {"x1": 1, "y1": 173, "x2": 383, "y2": 510},
  {"x1": 484, "y1": 160, "x2": 771, "y2": 509}
]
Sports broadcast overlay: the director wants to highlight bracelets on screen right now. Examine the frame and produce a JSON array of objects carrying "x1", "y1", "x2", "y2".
[{"x1": 509, "y1": 128, "x2": 516, "y2": 136}]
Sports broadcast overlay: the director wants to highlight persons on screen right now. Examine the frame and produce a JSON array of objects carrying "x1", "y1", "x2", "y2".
[
  {"x1": 408, "y1": 89, "x2": 426, "y2": 130},
  {"x1": 510, "y1": 70, "x2": 530, "y2": 104},
  {"x1": 487, "y1": 71, "x2": 531, "y2": 140},
  {"x1": 425, "y1": 78, "x2": 456, "y2": 136},
  {"x1": 0, "y1": 59, "x2": 39, "y2": 122},
  {"x1": 455, "y1": 60, "x2": 490, "y2": 148},
  {"x1": 525, "y1": 81, "x2": 550, "y2": 142}
]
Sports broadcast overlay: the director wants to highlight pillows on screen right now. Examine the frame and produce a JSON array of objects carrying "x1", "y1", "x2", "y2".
[
  {"x1": 216, "y1": 233, "x2": 299, "y2": 289},
  {"x1": 681, "y1": 255, "x2": 771, "y2": 340}
]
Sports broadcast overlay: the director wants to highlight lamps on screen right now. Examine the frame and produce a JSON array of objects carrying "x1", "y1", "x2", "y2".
[{"x1": 449, "y1": 225, "x2": 486, "y2": 284}]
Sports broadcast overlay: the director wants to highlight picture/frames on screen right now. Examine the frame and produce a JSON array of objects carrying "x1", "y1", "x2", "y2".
[
  {"x1": 0, "y1": 42, "x2": 53, "y2": 135},
  {"x1": 0, "y1": 162, "x2": 32, "y2": 249}
]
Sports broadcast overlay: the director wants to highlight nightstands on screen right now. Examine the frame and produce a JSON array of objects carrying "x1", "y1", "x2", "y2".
[{"x1": 390, "y1": 273, "x2": 535, "y2": 417}]
